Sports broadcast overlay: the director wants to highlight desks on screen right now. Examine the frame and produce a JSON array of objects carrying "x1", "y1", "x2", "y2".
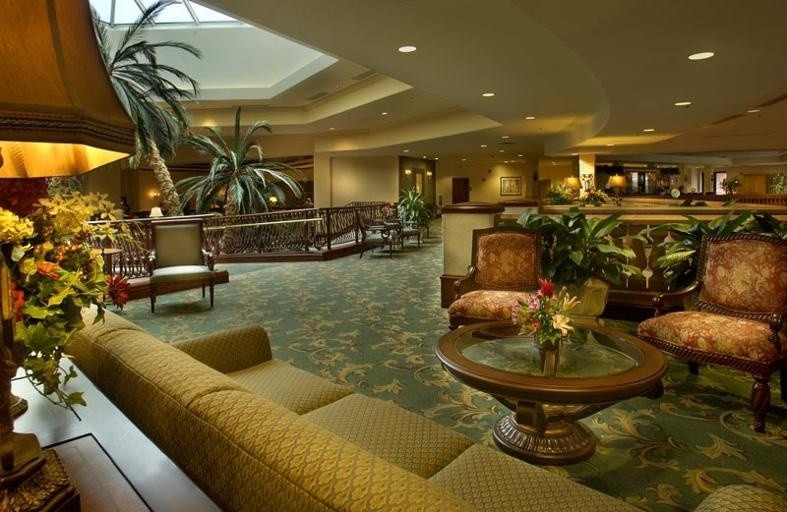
[{"x1": 11, "y1": 346, "x2": 224, "y2": 512}]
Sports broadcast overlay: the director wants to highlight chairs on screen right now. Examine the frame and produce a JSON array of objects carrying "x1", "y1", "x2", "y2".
[
  {"x1": 448, "y1": 226, "x2": 543, "y2": 331},
  {"x1": 354, "y1": 208, "x2": 429, "y2": 258},
  {"x1": 145, "y1": 219, "x2": 214, "y2": 313},
  {"x1": 636, "y1": 234, "x2": 787, "y2": 432}
]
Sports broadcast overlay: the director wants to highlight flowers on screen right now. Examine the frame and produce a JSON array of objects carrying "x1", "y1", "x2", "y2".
[
  {"x1": 580, "y1": 189, "x2": 610, "y2": 206},
  {"x1": 512, "y1": 278, "x2": 581, "y2": 344},
  {"x1": 0, "y1": 191, "x2": 132, "y2": 408}
]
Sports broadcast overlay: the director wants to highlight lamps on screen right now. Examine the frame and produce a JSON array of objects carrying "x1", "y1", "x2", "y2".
[
  {"x1": 0, "y1": 0, "x2": 135, "y2": 512},
  {"x1": 562, "y1": 175, "x2": 582, "y2": 194},
  {"x1": 149, "y1": 206, "x2": 164, "y2": 217},
  {"x1": 605, "y1": 173, "x2": 629, "y2": 206}
]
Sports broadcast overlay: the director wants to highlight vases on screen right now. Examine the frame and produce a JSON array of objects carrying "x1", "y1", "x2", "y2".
[
  {"x1": 0, "y1": 362, "x2": 28, "y2": 416},
  {"x1": 534, "y1": 331, "x2": 559, "y2": 349}
]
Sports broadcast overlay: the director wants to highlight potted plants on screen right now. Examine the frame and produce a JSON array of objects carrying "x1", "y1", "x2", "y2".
[
  {"x1": 640, "y1": 209, "x2": 787, "y2": 310},
  {"x1": 398, "y1": 186, "x2": 432, "y2": 243},
  {"x1": 517, "y1": 207, "x2": 646, "y2": 327}
]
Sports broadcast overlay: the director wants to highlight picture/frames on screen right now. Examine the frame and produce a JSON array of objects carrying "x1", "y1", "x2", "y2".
[{"x1": 500, "y1": 177, "x2": 522, "y2": 196}]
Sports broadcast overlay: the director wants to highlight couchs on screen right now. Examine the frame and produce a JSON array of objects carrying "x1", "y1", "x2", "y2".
[{"x1": 63, "y1": 302, "x2": 787, "y2": 512}]
